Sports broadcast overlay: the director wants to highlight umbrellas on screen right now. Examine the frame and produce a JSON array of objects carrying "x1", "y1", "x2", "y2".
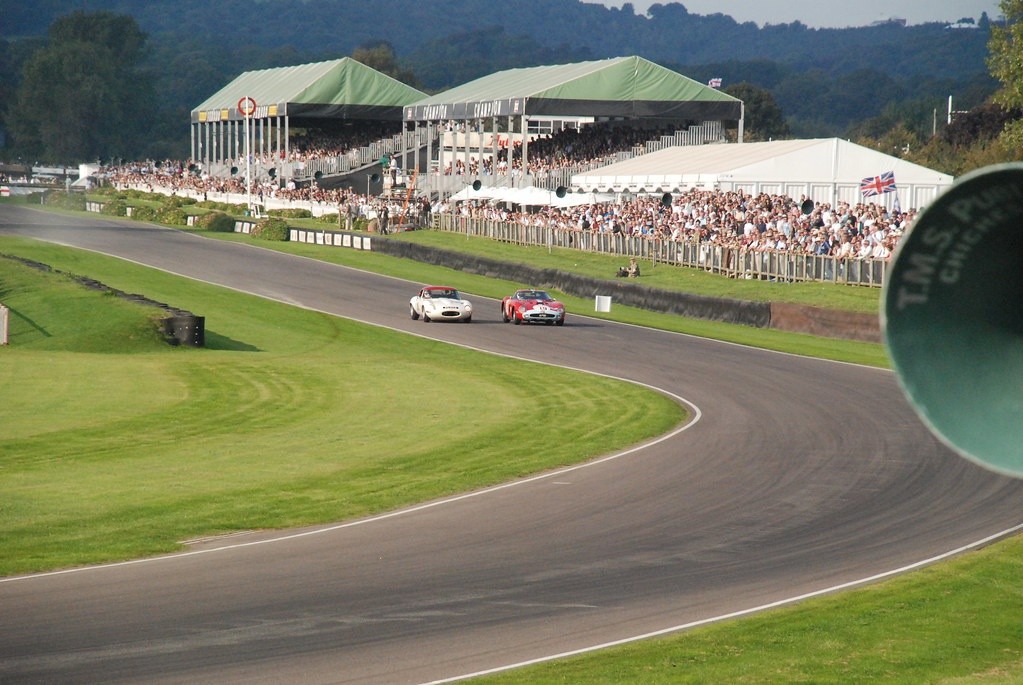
[{"x1": 449, "y1": 186, "x2": 610, "y2": 209}]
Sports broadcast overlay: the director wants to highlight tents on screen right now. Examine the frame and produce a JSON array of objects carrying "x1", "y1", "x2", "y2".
[
  {"x1": 403, "y1": 56, "x2": 745, "y2": 185},
  {"x1": 191, "y1": 57, "x2": 431, "y2": 165}
]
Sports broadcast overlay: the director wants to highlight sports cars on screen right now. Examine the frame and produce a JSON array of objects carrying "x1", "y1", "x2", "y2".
[
  {"x1": 501, "y1": 289, "x2": 565, "y2": 327},
  {"x1": 409, "y1": 286, "x2": 473, "y2": 323}
]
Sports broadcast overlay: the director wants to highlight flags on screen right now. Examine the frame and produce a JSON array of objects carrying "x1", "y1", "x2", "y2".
[{"x1": 860, "y1": 171, "x2": 896, "y2": 198}]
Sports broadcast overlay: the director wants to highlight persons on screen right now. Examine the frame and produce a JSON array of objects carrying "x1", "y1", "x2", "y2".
[
  {"x1": 442, "y1": 190, "x2": 926, "y2": 283},
  {"x1": 216, "y1": 120, "x2": 433, "y2": 164},
  {"x1": 93, "y1": 158, "x2": 312, "y2": 203},
  {"x1": 0, "y1": 173, "x2": 57, "y2": 184},
  {"x1": 388, "y1": 155, "x2": 398, "y2": 187},
  {"x1": 435, "y1": 121, "x2": 699, "y2": 181},
  {"x1": 627, "y1": 258, "x2": 640, "y2": 277},
  {"x1": 312, "y1": 181, "x2": 431, "y2": 236}
]
